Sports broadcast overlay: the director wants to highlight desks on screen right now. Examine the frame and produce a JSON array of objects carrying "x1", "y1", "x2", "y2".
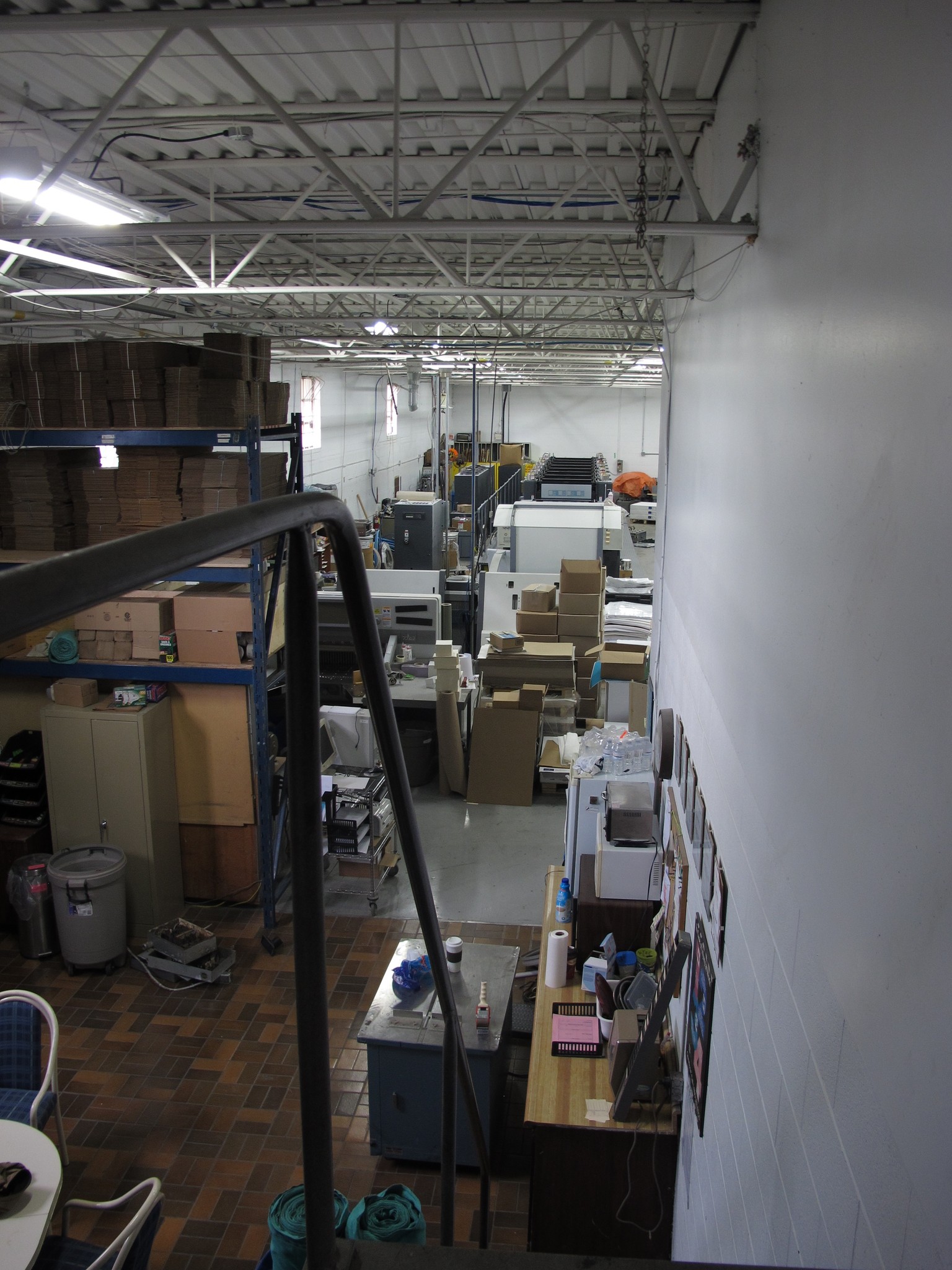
[
  {"x1": 0, "y1": 1118, "x2": 62, "y2": 1270},
  {"x1": 523, "y1": 865, "x2": 682, "y2": 1259},
  {"x1": 353, "y1": 676, "x2": 480, "y2": 781}
]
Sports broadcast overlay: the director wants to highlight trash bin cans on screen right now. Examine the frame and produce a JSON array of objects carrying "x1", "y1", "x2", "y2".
[
  {"x1": 11, "y1": 853, "x2": 59, "y2": 961},
  {"x1": 48, "y1": 845, "x2": 129, "y2": 966}
]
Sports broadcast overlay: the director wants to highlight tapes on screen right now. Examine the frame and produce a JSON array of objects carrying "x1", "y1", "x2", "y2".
[
  {"x1": 396, "y1": 654, "x2": 406, "y2": 663},
  {"x1": 414, "y1": 662, "x2": 425, "y2": 667}
]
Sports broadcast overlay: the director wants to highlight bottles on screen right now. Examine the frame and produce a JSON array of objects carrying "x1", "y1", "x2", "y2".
[
  {"x1": 401, "y1": 644, "x2": 412, "y2": 661},
  {"x1": 608, "y1": 492, "x2": 613, "y2": 502},
  {"x1": 600, "y1": 736, "x2": 653, "y2": 776},
  {"x1": 555, "y1": 878, "x2": 572, "y2": 924}
]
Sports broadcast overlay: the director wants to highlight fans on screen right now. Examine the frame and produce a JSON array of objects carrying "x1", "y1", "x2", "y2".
[{"x1": 453, "y1": 454, "x2": 468, "y2": 472}]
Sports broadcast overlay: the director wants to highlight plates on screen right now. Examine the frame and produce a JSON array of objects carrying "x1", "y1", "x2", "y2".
[{"x1": 613, "y1": 971, "x2": 656, "y2": 1010}]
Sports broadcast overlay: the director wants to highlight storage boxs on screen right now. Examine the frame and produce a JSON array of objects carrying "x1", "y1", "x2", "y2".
[
  {"x1": 581, "y1": 932, "x2": 617, "y2": 992},
  {"x1": 466, "y1": 557, "x2": 653, "y2": 805},
  {"x1": 1, "y1": 590, "x2": 251, "y2": 708},
  {"x1": 338, "y1": 837, "x2": 399, "y2": 878},
  {"x1": 500, "y1": 444, "x2": 525, "y2": 466},
  {"x1": 452, "y1": 517, "x2": 472, "y2": 531},
  {"x1": 457, "y1": 504, "x2": 472, "y2": 513},
  {"x1": 392, "y1": 640, "x2": 463, "y2": 701},
  {"x1": 423, "y1": 466, "x2": 442, "y2": 477},
  {"x1": 629, "y1": 502, "x2": 657, "y2": 521}
]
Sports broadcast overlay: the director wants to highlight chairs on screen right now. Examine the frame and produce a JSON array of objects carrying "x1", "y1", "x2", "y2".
[
  {"x1": 0, "y1": 990, "x2": 70, "y2": 1164},
  {"x1": 30, "y1": 1176, "x2": 166, "y2": 1270}
]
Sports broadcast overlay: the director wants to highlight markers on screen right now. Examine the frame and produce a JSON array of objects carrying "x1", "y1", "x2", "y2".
[{"x1": 661, "y1": 1032, "x2": 671, "y2": 1046}]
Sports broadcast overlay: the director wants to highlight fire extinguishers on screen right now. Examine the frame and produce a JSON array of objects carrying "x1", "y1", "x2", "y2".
[{"x1": 373, "y1": 515, "x2": 379, "y2": 531}]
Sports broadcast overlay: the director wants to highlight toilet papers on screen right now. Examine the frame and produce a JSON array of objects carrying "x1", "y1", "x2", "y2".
[{"x1": 544, "y1": 929, "x2": 569, "y2": 989}]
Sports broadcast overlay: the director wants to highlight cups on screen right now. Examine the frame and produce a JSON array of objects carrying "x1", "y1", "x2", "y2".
[
  {"x1": 636, "y1": 948, "x2": 657, "y2": 975},
  {"x1": 566, "y1": 946, "x2": 577, "y2": 980},
  {"x1": 615, "y1": 951, "x2": 637, "y2": 979},
  {"x1": 446, "y1": 936, "x2": 463, "y2": 973}
]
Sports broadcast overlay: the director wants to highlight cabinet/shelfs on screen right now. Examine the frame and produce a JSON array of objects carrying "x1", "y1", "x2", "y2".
[
  {"x1": 0, "y1": 413, "x2": 307, "y2": 952},
  {"x1": 451, "y1": 442, "x2": 530, "y2": 498},
  {"x1": 0, "y1": 729, "x2": 48, "y2": 932},
  {"x1": 40, "y1": 695, "x2": 184, "y2": 947},
  {"x1": 357, "y1": 937, "x2": 520, "y2": 1168}
]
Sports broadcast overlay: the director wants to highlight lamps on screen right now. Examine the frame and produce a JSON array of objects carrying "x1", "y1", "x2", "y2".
[
  {"x1": 0, "y1": 156, "x2": 173, "y2": 227},
  {"x1": 495, "y1": 432, "x2": 502, "y2": 440}
]
